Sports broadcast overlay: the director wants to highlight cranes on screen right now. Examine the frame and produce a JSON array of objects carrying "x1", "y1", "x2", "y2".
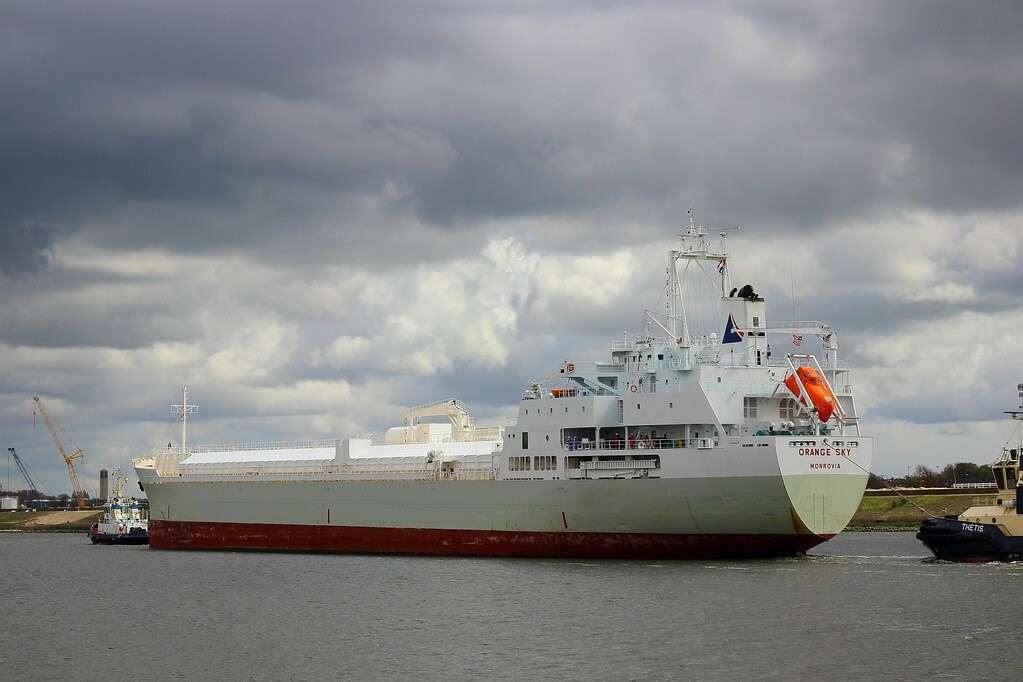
[
  {"x1": 6, "y1": 447, "x2": 57, "y2": 510},
  {"x1": 32, "y1": 396, "x2": 86, "y2": 511}
]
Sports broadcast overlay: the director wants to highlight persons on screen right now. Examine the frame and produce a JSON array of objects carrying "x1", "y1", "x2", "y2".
[
  {"x1": 614, "y1": 433, "x2": 618, "y2": 447},
  {"x1": 643, "y1": 434, "x2": 650, "y2": 446},
  {"x1": 628, "y1": 433, "x2": 636, "y2": 449},
  {"x1": 86, "y1": 501, "x2": 103, "y2": 506}
]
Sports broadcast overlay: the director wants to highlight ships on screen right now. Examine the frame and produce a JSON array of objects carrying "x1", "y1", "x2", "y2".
[{"x1": 132, "y1": 205, "x2": 874, "y2": 559}]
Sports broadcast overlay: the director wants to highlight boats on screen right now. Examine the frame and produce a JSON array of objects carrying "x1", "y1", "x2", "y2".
[
  {"x1": 913, "y1": 383, "x2": 1023, "y2": 562},
  {"x1": 87, "y1": 437, "x2": 150, "y2": 546},
  {"x1": 550, "y1": 388, "x2": 576, "y2": 398},
  {"x1": 785, "y1": 366, "x2": 834, "y2": 423}
]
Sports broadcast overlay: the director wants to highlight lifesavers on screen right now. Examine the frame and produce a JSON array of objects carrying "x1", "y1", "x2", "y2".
[
  {"x1": 91, "y1": 535, "x2": 99, "y2": 542},
  {"x1": 638, "y1": 442, "x2": 644, "y2": 448},
  {"x1": 567, "y1": 364, "x2": 574, "y2": 371},
  {"x1": 631, "y1": 385, "x2": 637, "y2": 392}
]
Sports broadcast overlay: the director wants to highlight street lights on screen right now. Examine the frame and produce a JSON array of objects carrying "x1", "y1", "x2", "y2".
[
  {"x1": 907, "y1": 465, "x2": 910, "y2": 476},
  {"x1": 936, "y1": 465, "x2": 939, "y2": 485}
]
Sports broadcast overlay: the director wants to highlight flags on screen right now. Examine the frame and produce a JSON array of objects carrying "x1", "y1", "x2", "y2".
[
  {"x1": 716, "y1": 260, "x2": 723, "y2": 273},
  {"x1": 792, "y1": 335, "x2": 802, "y2": 346}
]
